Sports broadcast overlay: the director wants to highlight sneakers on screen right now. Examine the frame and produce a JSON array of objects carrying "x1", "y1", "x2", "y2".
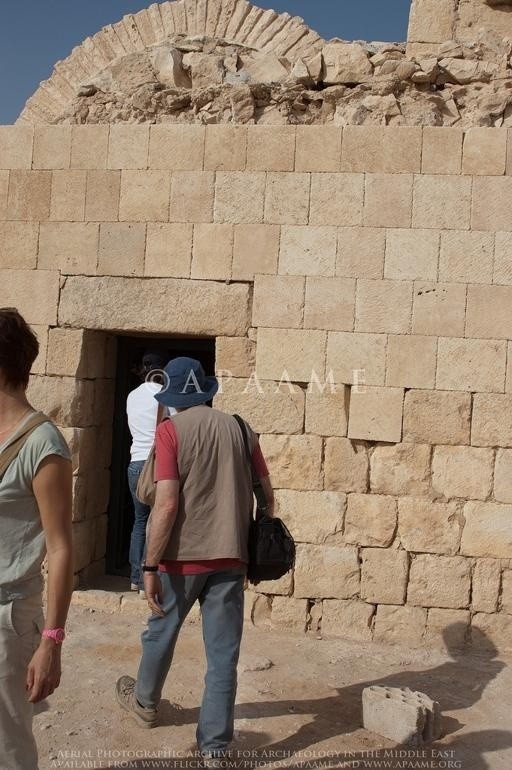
[
  {"x1": 117, "y1": 676, "x2": 158, "y2": 729},
  {"x1": 203, "y1": 752, "x2": 225, "y2": 768}
]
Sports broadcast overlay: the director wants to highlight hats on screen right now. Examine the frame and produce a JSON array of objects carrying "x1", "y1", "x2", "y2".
[{"x1": 154, "y1": 356, "x2": 218, "y2": 407}]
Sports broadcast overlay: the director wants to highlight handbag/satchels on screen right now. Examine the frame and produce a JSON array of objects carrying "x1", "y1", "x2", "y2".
[
  {"x1": 135, "y1": 445, "x2": 159, "y2": 505},
  {"x1": 248, "y1": 517, "x2": 296, "y2": 581}
]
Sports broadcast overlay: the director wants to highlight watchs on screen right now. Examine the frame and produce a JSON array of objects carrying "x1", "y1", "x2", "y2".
[
  {"x1": 140, "y1": 560, "x2": 158, "y2": 574},
  {"x1": 43, "y1": 628, "x2": 65, "y2": 645}
]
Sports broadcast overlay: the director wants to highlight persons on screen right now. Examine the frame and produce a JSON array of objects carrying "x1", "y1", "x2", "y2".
[
  {"x1": 0, "y1": 306, "x2": 74, "y2": 770},
  {"x1": 114, "y1": 356, "x2": 274, "y2": 769},
  {"x1": 126, "y1": 353, "x2": 178, "y2": 601}
]
